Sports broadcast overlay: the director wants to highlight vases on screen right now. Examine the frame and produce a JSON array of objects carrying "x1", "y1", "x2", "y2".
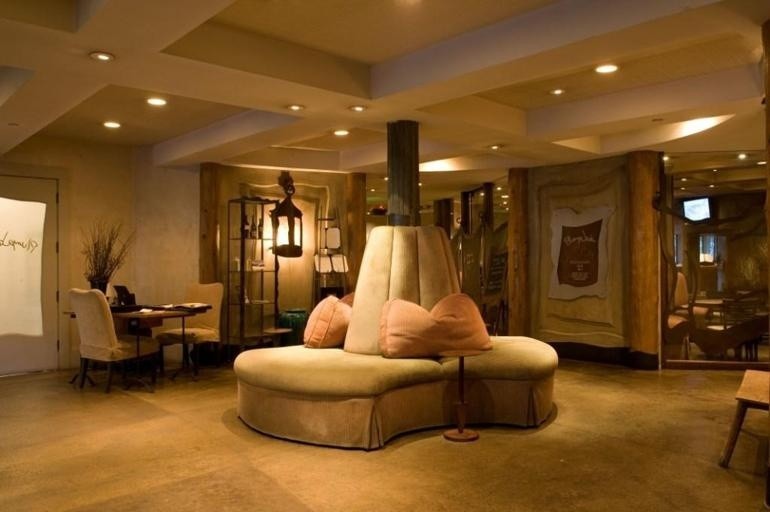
[{"x1": 278, "y1": 308, "x2": 310, "y2": 347}]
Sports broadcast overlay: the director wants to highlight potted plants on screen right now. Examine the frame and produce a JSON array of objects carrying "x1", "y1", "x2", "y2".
[{"x1": 78, "y1": 213, "x2": 135, "y2": 296}]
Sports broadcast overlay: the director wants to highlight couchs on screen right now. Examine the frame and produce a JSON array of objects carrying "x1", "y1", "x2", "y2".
[{"x1": 232, "y1": 225, "x2": 558, "y2": 452}]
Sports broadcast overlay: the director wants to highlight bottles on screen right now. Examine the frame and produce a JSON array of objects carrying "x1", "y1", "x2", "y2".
[
  {"x1": 244, "y1": 215, "x2": 264, "y2": 240},
  {"x1": 232, "y1": 255, "x2": 266, "y2": 271}
]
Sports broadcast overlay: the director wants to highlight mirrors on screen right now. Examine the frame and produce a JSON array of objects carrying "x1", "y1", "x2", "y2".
[{"x1": 659, "y1": 150, "x2": 770, "y2": 371}]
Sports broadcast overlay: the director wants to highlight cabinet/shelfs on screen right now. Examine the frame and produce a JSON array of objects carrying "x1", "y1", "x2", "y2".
[{"x1": 226, "y1": 197, "x2": 279, "y2": 364}]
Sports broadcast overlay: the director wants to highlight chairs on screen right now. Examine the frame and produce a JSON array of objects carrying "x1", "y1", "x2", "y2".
[
  {"x1": 67, "y1": 287, "x2": 161, "y2": 392},
  {"x1": 706, "y1": 299, "x2": 759, "y2": 361}
]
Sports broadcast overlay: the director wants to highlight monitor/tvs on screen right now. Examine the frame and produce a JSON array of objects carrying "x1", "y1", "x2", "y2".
[{"x1": 679, "y1": 196, "x2": 717, "y2": 226}]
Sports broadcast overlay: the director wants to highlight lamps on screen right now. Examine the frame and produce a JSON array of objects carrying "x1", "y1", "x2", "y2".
[{"x1": 269, "y1": 176, "x2": 303, "y2": 257}]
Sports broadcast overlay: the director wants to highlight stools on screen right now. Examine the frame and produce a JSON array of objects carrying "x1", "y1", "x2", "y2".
[{"x1": 158, "y1": 327, "x2": 217, "y2": 376}]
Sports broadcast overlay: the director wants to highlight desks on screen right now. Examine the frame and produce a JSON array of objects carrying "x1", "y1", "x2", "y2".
[
  {"x1": 63, "y1": 305, "x2": 196, "y2": 393},
  {"x1": 688, "y1": 297, "x2": 725, "y2": 351}
]
